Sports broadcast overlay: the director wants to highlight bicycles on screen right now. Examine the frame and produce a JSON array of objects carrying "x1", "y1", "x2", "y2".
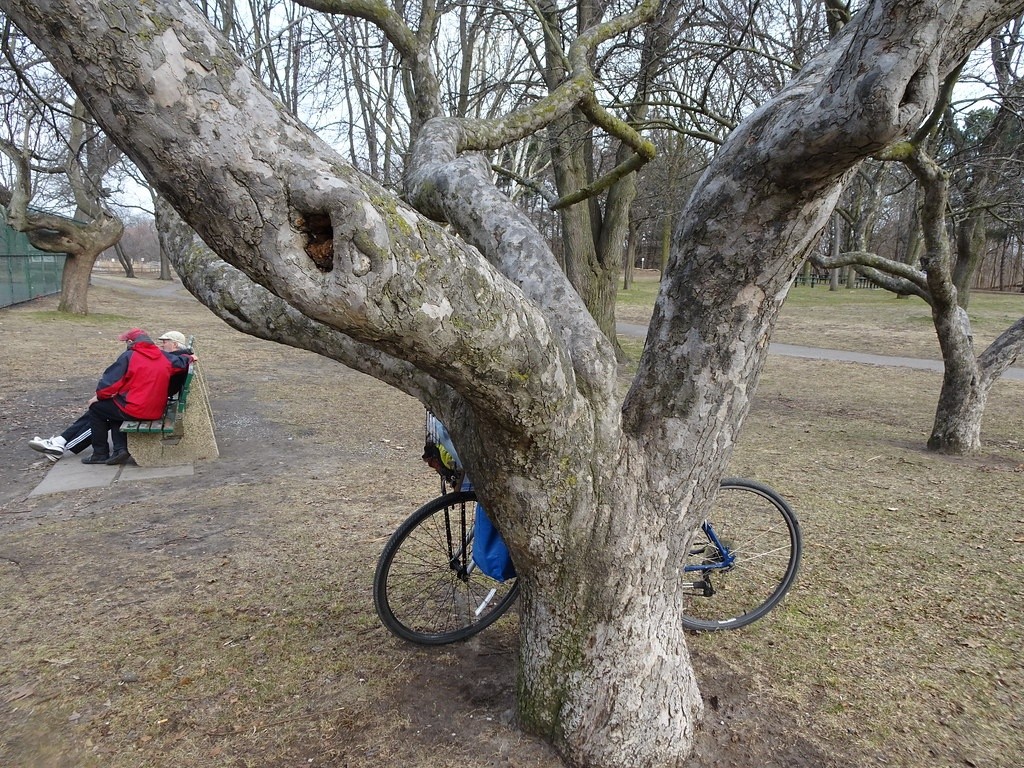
[{"x1": 374, "y1": 406, "x2": 804, "y2": 649}]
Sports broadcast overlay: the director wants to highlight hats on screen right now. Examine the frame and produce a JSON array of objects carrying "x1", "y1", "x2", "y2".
[
  {"x1": 119, "y1": 329, "x2": 147, "y2": 341},
  {"x1": 159, "y1": 331, "x2": 185, "y2": 344}
]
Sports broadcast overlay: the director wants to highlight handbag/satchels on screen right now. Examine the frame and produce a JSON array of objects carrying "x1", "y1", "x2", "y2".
[{"x1": 472, "y1": 504, "x2": 519, "y2": 582}]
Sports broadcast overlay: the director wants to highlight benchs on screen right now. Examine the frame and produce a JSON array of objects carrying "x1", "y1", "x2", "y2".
[{"x1": 118, "y1": 335, "x2": 195, "y2": 447}]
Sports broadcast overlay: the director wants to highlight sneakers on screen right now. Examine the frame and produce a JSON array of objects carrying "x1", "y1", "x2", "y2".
[
  {"x1": 29, "y1": 439, "x2": 64, "y2": 455},
  {"x1": 33, "y1": 437, "x2": 62, "y2": 462}
]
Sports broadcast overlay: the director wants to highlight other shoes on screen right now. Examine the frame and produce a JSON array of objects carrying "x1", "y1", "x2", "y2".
[
  {"x1": 105, "y1": 448, "x2": 129, "y2": 464},
  {"x1": 81, "y1": 449, "x2": 109, "y2": 464}
]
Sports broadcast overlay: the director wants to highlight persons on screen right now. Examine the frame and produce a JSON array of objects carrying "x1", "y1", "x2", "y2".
[
  {"x1": 28, "y1": 327, "x2": 192, "y2": 461},
  {"x1": 86, "y1": 325, "x2": 171, "y2": 466}
]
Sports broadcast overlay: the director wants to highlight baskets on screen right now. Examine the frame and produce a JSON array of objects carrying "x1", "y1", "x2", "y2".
[{"x1": 423, "y1": 406, "x2": 478, "y2": 494}]
